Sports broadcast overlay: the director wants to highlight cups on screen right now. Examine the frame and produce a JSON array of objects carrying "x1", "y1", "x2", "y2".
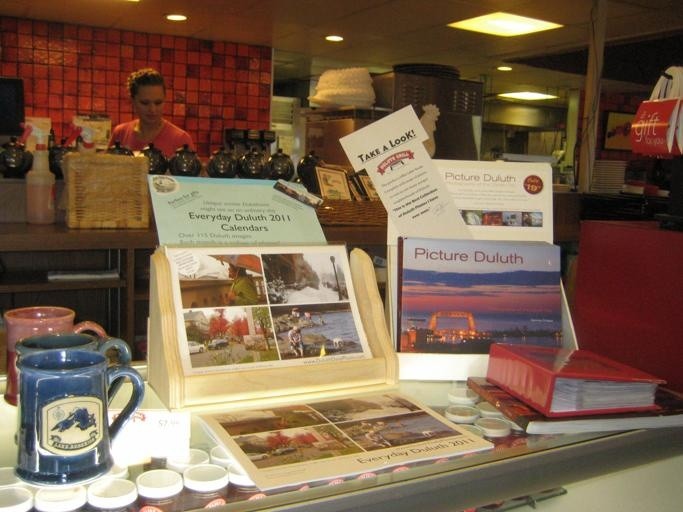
[
  {"x1": 4, "y1": 307, "x2": 144, "y2": 486},
  {"x1": 624, "y1": 180, "x2": 670, "y2": 196}
]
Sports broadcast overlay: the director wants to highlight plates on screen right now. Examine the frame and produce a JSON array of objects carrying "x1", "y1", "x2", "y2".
[{"x1": 589, "y1": 159, "x2": 628, "y2": 194}]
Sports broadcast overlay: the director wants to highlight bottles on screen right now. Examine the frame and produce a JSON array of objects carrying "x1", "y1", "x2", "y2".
[
  {"x1": 297, "y1": 150, "x2": 325, "y2": 181},
  {"x1": 104, "y1": 141, "x2": 134, "y2": 156},
  {"x1": 205, "y1": 145, "x2": 238, "y2": 178},
  {"x1": 560, "y1": 136, "x2": 567, "y2": 152},
  {"x1": 25, "y1": 144, "x2": 56, "y2": 225},
  {"x1": 268, "y1": 147, "x2": 295, "y2": 180},
  {"x1": 140, "y1": 143, "x2": 168, "y2": 175},
  {"x1": 1, "y1": 137, "x2": 34, "y2": 181},
  {"x1": 552, "y1": 164, "x2": 577, "y2": 193},
  {"x1": 168, "y1": 143, "x2": 202, "y2": 177},
  {"x1": 48, "y1": 144, "x2": 77, "y2": 181},
  {"x1": 237, "y1": 147, "x2": 268, "y2": 179}
]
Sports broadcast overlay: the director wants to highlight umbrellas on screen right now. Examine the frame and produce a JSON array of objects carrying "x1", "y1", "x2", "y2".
[{"x1": 209, "y1": 254, "x2": 261, "y2": 291}]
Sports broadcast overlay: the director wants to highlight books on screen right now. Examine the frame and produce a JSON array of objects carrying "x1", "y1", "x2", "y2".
[
  {"x1": 488, "y1": 342, "x2": 667, "y2": 419},
  {"x1": 396, "y1": 235, "x2": 562, "y2": 353},
  {"x1": 466, "y1": 376, "x2": 682, "y2": 435}
]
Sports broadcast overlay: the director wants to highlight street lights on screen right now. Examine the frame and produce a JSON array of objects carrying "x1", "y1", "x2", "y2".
[{"x1": 330, "y1": 255, "x2": 343, "y2": 301}]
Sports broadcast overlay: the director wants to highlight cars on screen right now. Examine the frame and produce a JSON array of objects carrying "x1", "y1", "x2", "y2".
[
  {"x1": 246, "y1": 452, "x2": 269, "y2": 462},
  {"x1": 207, "y1": 339, "x2": 229, "y2": 350},
  {"x1": 272, "y1": 447, "x2": 295, "y2": 456},
  {"x1": 188, "y1": 341, "x2": 205, "y2": 354}
]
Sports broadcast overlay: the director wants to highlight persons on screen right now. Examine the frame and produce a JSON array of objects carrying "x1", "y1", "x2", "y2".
[
  {"x1": 286, "y1": 326, "x2": 303, "y2": 356},
  {"x1": 105, "y1": 69, "x2": 194, "y2": 160},
  {"x1": 224, "y1": 264, "x2": 258, "y2": 305}
]
